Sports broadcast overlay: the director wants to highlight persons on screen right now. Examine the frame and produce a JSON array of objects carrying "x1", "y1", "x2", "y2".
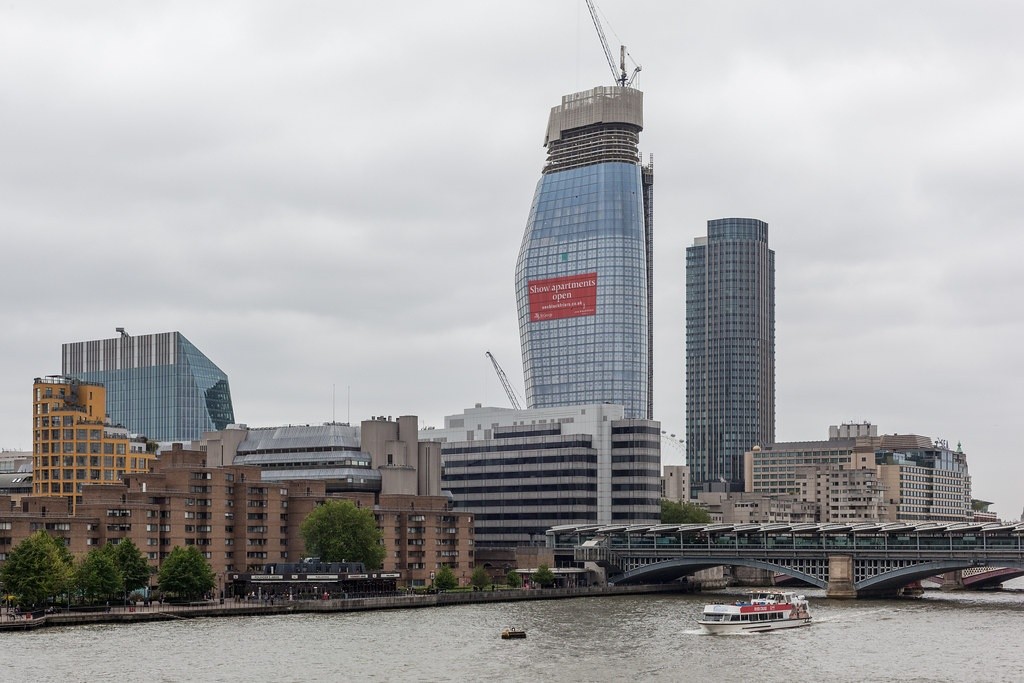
[
  {"x1": 9, "y1": 611, "x2": 15, "y2": 620},
  {"x1": 133, "y1": 601, "x2": 136, "y2": 605},
  {"x1": 50, "y1": 605, "x2": 58, "y2": 613},
  {"x1": 270, "y1": 594, "x2": 275, "y2": 606},
  {"x1": 159, "y1": 597, "x2": 162, "y2": 603},
  {"x1": 149, "y1": 596, "x2": 152, "y2": 604},
  {"x1": 251, "y1": 591, "x2": 255, "y2": 599},
  {"x1": 161, "y1": 594, "x2": 163, "y2": 599},
  {"x1": 15, "y1": 607, "x2": 20, "y2": 615},
  {"x1": 264, "y1": 593, "x2": 268, "y2": 600},
  {"x1": 10, "y1": 599, "x2": 13, "y2": 610}
]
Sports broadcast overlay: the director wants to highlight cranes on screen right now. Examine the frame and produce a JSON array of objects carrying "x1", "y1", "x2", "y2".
[
  {"x1": 585, "y1": 0, "x2": 643, "y2": 88},
  {"x1": 485, "y1": 351, "x2": 527, "y2": 411}
]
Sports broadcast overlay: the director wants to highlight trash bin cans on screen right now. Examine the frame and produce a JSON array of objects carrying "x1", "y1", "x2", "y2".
[{"x1": 129, "y1": 600, "x2": 134, "y2": 612}]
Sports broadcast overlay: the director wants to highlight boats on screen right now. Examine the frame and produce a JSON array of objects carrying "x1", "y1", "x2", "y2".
[
  {"x1": 697, "y1": 590, "x2": 812, "y2": 635},
  {"x1": 502, "y1": 628, "x2": 526, "y2": 639}
]
